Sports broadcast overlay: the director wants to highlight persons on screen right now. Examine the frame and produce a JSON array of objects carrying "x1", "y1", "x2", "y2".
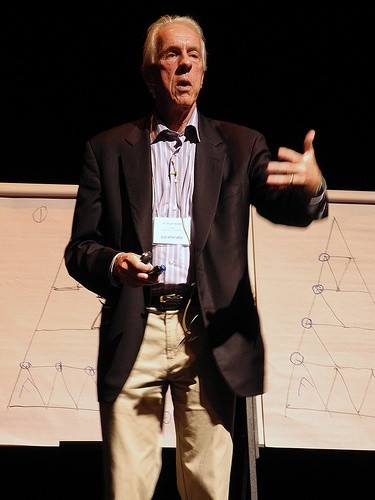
[{"x1": 64, "y1": 16, "x2": 329, "y2": 500}]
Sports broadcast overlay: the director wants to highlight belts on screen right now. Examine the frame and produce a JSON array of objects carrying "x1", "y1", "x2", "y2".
[{"x1": 145, "y1": 293, "x2": 190, "y2": 311}]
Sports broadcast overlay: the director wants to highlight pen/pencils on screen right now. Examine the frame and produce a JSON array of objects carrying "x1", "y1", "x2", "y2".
[{"x1": 136, "y1": 265, "x2": 166, "y2": 286}]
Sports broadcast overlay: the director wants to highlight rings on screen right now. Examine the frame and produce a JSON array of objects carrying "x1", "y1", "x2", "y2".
[{"x1": 290, "y1": 174, "x2": 294, "y2": 184}]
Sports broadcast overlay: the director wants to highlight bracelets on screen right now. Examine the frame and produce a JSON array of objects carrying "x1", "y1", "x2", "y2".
[{"x1": 315, "y1": 176, "x2": 323, "y2": 197}]
